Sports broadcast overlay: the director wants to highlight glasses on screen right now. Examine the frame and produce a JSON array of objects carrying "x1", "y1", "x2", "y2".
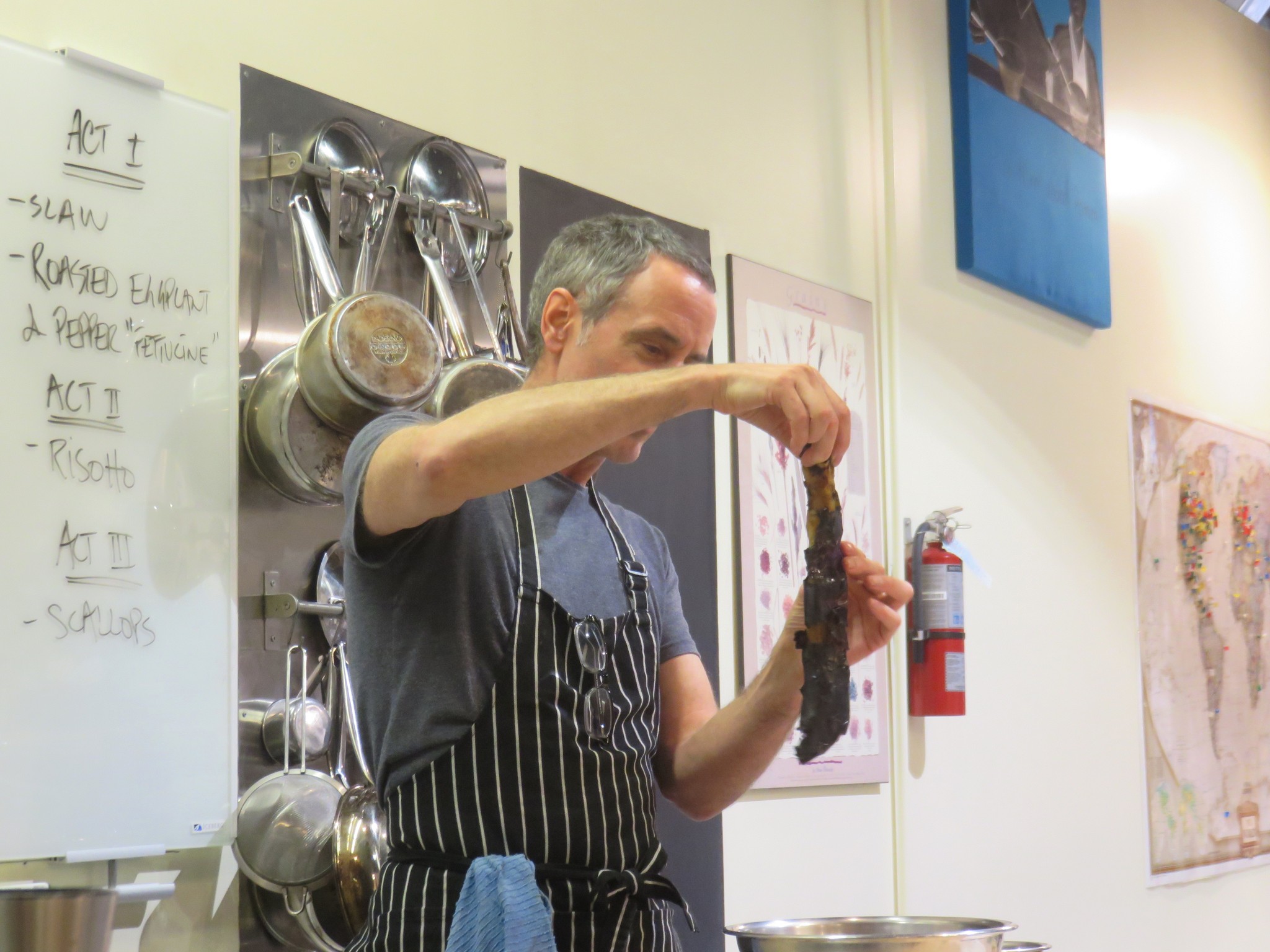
[{"x1": 574, "y1": 613, "x2": 613, "y2": 745}]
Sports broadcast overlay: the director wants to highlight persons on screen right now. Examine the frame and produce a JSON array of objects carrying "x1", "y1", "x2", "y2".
[{"x1": 339, "y1": 214, "x2": 913, "y2": 952}]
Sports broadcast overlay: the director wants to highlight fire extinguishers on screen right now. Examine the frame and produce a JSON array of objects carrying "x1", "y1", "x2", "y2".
[{"x1": 906, "y1": 506, "x2": 966, "y2": 717}]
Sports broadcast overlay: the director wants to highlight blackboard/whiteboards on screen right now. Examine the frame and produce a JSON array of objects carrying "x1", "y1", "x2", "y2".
[{"x1": 0, "y1": 32, "x2": 239, "y2": 863}]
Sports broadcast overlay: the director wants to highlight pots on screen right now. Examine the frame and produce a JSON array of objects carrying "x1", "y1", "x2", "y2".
[
  {"x1": 230, "y1": 190, "x2": 528, "y2": 952},
  {"x1": 0, "y1": 886, "x2": 178, "y2": 952}
]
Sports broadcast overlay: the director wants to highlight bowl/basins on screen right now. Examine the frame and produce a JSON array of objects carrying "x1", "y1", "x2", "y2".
[
  {"x1": 723, "y1": 916, "x2": 1016, "y2": 952},
  {"x1": 1002, "y1": 941, "x2": 1053, "y2": 952}
]
